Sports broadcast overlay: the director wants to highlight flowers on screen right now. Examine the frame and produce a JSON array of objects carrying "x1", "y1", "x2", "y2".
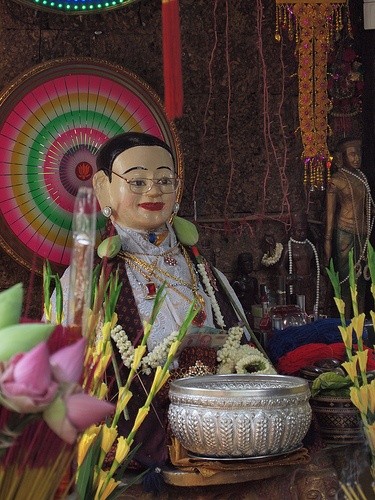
[
  {"x1": 215, "y1": 320, "x2": 276, "y2": 375},
  {"x1": 326, "y1": 239, "x2": 375, "y2": 455},
  {"x1": 0, "y1": 264, "x2": 201, "y2": 500}
]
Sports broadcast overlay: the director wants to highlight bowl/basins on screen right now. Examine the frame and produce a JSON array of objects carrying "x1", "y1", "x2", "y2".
[{"x1": 167, "y1": 374, "x2": 312, "y2": 458}]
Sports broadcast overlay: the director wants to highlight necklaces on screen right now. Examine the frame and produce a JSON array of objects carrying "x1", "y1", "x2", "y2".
[
  {"x1": 103, "y1": 243, "x2": 225, "y2": 406},
  {"x1": 340, "y1": 167, "x2": 375, "y2": 285},
  {"x1": 288, "y1": 237, "x2": 320, "y2": 323}
]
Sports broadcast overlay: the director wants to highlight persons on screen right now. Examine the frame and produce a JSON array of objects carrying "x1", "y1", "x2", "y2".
[
  {"x1": 41, "y1": 133, "x2": 276, "y2": 472},
  {"x1": 231, "y1": 250, "x2": 262, "y2": 327},
  {"x1": 325, "y1": 138, "x2": 375, "y2": 324},
  {"x1": 263, "y1": 209, "x2": 328, "y2": 321}
]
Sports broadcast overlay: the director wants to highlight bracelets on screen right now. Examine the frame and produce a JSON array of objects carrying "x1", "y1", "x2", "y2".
[{"x1": 260, "y1": 243, "x2": 284, "y2": 266}]
